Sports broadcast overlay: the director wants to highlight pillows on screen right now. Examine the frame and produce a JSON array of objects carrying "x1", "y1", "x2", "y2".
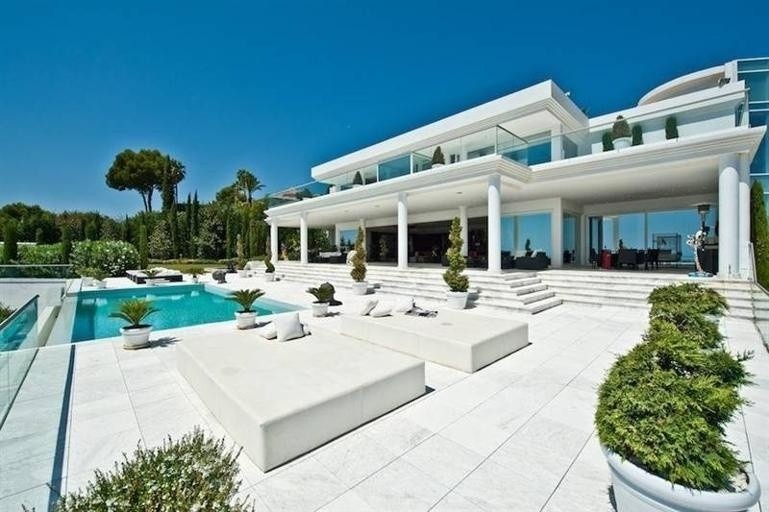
[
  {"x1": 271, "y1": 312, "x2": 305, "y2": 342},
  {"x1": 368, "y1": 304, "x2": 392, "y2": 317},
  {"x1": 257, "y1": 322, "x2": 278, "y2": 339},
  {"x1": 359, "y1": 298, "x2": 377, "y2": 317}
]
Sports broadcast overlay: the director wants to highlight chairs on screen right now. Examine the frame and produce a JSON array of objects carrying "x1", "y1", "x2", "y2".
[
  {"x1": 588, "y1": 243, "x2": 683, "y2": 270},
  {"x1": 301, "y1": 243, "x2": 349, "y2": 264},
  {"x1": 407, "y1": 247, "x2": 550, "y2": 271}
]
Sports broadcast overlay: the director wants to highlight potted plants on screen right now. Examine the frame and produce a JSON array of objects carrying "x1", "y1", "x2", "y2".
[
  {"x1": 90, "y1": 263, "x2": 111, "y2": 289},
  {"x1": 349, "y1": 225, "x2": 369, "y2": 295},
  {"x1": 188, "y1": 263, "x2": 205, "y2": 283},
  {"x1": 237, "y1": 258, "x2": 249, "y2": 278},
  {"x1": 139, "y1": 263, "x2": 163, "y2": 286},
  {"x1": 218, "y1": 258, "x2": 240, "y2": 282},
  {"x1": 352, "y1": 172, "x2": 363, "y2": 189},
  {"x1": 224, "y1": 286, "x2": 267, "y2": 328},
  {"x1": 431, "y1": 146, "x2": 446, "y2": 168},
  {"x1": 263, "y1": 253, "x2": 277, "y2": 282},
  {"x1": 105, "y1": 295, "x2": 162, "y2": 350},
  {"x1": 594, "y1": 313, "x2": 761, "y2": 511},
  {"x1": 74, "y1": 266, "x2": 94, "y2": 286},
  {"x1": 611, "y1": 116, "x2": 633, "y2": 149},
  {"x1": 442, "y1": 216, "x2": 470, "y2": 310},
  {"x1": 305, "y1": 283, "x2": 336, "y2": 318}
]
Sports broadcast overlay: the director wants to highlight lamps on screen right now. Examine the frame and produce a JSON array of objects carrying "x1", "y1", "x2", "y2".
[{"x1": 687, "y1": 203, "x2": 719, "y2": 279}]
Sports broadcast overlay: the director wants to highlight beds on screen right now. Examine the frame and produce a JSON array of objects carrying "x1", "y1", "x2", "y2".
[
  {"x1": 176, "y1": 322, "x2": 427, "y2": 471},
  {"x1": 124, "y1": 266, "x2": 183, "y2": 285},
  {"x1": 341, "y1": 303, "x2": 528, "y2": 373}
]
